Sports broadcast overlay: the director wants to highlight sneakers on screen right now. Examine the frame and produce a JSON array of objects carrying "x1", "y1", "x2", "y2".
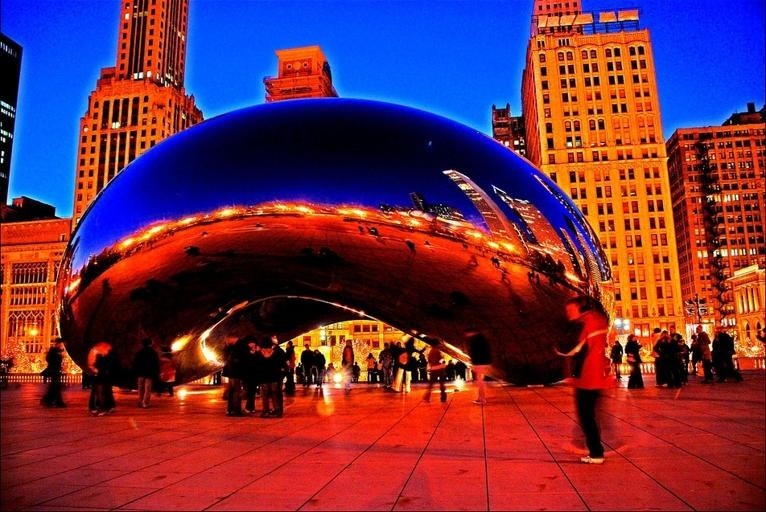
[{"x1": 582, "y1": 457, "x2": 604, "y2": 464}]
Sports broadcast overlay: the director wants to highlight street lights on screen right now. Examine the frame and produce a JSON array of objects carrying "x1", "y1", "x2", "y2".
[{"x1": 684, "y1": 292, "x2": 707, "y2": 327}]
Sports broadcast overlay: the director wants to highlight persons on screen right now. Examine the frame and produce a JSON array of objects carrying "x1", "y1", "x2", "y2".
[
  {"x1": 328, "y1": 336, "x2": 468, "y2": 403},
  {"x1": 61, "y1": 212, "x2": 575, "y2": 371},
  {"x1": 463, "y1": 327, "x2": 494, "y2": 405},
  {"x1": 39, "y1": 337, "x2": 68, "y2": 408},
  {"x1": 221, "y1": 333, "x2": 327, "y2": 419},
  {"x1": 609, "y1": 325, "x2": 744, "y2": 390},
  {"x1": 87, "y1": 337, "x2": 178, "y2": 416},
  {"x1": 550, "y1": 295, "x2": 618, "y2": 465}
]
